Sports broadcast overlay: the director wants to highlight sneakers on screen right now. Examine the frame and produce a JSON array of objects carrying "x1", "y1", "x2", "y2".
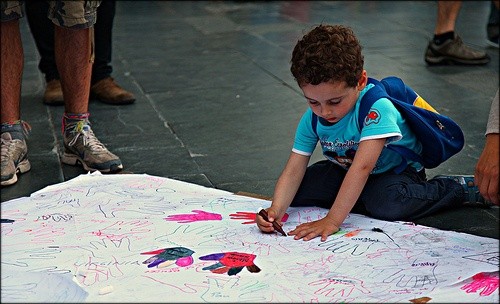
[
  {"x1": 427, "y1": 173, "x2": 489, "y2": 207},
  {"x1": 90, "y1": 76, "x2": 135, "y2": 104},
  {"x1": 0, "y1": 133, "x2": 30, "y2": 184},
  {"x1": 42, "y1": 79, "x2": 64, "y2": 105},
  {"x1": 424, "y1": 35, "x2": 491, "y2": 65},
  {"x1": 59, "y1": 121, "x2": 123, "y2": 172},
  {"x1": 486, "y1": 20, "x2": 500, "y2": 43}
]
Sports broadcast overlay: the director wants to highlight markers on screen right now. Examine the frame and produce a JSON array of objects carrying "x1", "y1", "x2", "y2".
[
  {"x1": 259, "y1": 209, "x2": 286, "y2": 236},
  {"x1": 0, "y1": 219, "x2": 14, "y2": 223}
]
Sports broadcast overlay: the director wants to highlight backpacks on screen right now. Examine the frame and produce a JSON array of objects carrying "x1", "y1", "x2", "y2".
[{"x1": 312, "y1": 77, "x2": 465, "y2": 176}]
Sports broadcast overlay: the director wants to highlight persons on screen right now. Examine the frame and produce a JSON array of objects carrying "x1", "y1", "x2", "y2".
[
  {"x1": 24, "y1": 0, "x2": 136, "y2": 105},
  {"x1": 474, "y1": 87, "x2": 500, "y2": 204},
  {"x1": 0, "y1": 0, "x2": 123, "y2": 185},
  {"x1": 424, "y1": 0, "x2": 490, "y2": 65},
  {"x1": 256, "y1": 25, "x2": 491, "y2": 241}
]
